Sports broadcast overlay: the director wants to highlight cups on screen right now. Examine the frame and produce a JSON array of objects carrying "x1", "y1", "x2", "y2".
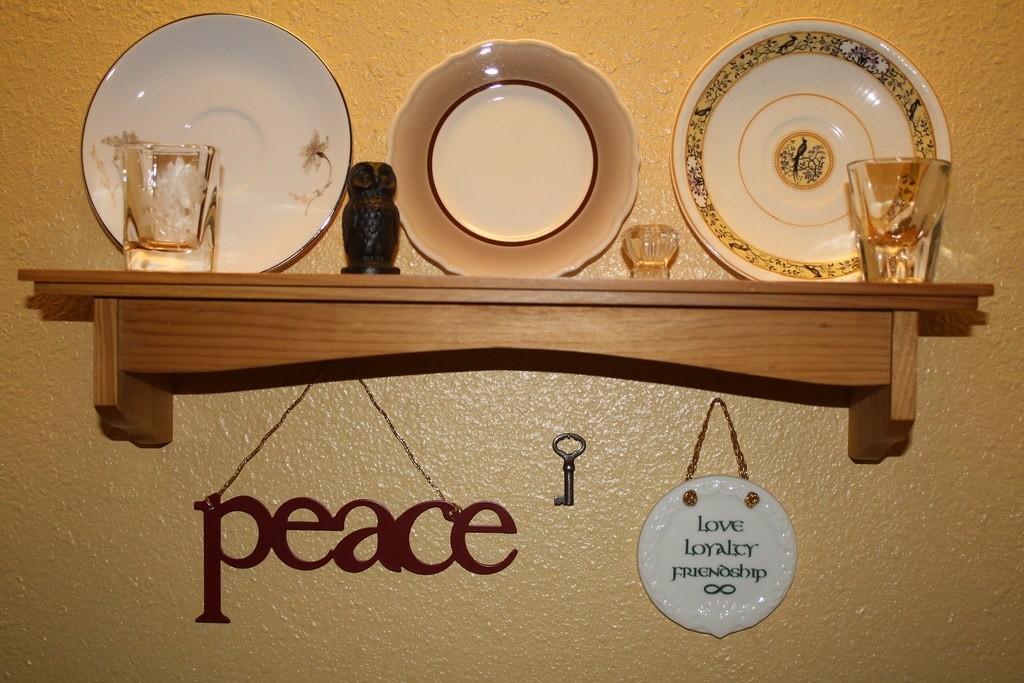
[
  {"x1": 121, "y1": 142, "x2": 219, "y2": 273},
  {"x1": 846, "y1": 156, "x2": 952, "y2": 284}
]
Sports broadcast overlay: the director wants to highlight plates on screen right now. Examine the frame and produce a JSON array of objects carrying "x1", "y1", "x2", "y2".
[
  {"x1": 81, "y1": 12, "x2": 353, "y2": 273},
  {"x1": 669, "y1": 17, "x2": 953, "y2": 283},
  {"x1": 383, "y1": 38, "x2": 641, "y2": 278}
]
